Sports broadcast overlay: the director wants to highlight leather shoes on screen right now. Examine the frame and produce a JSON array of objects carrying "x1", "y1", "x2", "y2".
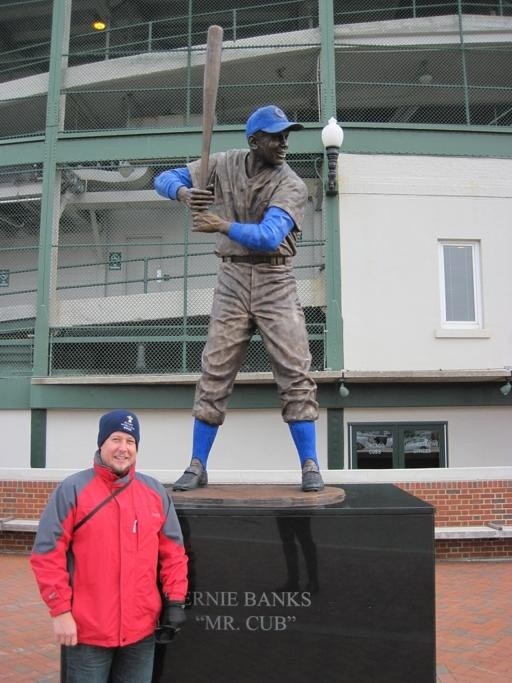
[
  {"x1": 301, "y1": 470, "x2": 325, "y2": 491},
  {"x1": 171, "y1": 464, "x2": 208, "y2": 492}
]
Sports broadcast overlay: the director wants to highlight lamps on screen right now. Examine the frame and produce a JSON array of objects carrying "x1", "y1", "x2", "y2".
[
  {"x1": 339, "y1": 382, "x2": 350, "y2": 398},
  {"x1": 499, "y1": 376, "x2": 512, "y2": 396}
]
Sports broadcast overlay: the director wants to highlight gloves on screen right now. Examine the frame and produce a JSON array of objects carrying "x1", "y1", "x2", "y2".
[{"x1": 159, "y1": 600, "x2": 187, "y2": 634}]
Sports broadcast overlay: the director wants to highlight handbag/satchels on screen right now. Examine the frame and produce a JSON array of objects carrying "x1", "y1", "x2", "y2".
[{"x1": 67, "y1": 541, "x2": 75, "y2": 586}]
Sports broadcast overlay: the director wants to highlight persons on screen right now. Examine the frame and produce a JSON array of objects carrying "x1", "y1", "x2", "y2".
[
  {"x1": 152, "y1": 104, "x2": 327, "y2": 493},
  {"x1": 154, "y1": 513, "x2": 197, "y2": 683},
  {"x1": 276, "y1": 515, "x2": 322, "y2": 598},
  {"x1": 29, "y1": 408, "x2": 190, "y2": 682}
]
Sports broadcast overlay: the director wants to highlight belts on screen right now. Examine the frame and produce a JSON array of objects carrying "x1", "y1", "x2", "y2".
[{"x1": 220, "y1": 255, "x2": 293, "y2": 264}]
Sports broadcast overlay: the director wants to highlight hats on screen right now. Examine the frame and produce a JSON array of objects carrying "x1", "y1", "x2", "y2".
[
  {"x1": 245, "y1": 104, "x2": 304, "y2": 139},
  {"x1": 97, "y1": 410, "x2": 140, "y2": 448}
]
